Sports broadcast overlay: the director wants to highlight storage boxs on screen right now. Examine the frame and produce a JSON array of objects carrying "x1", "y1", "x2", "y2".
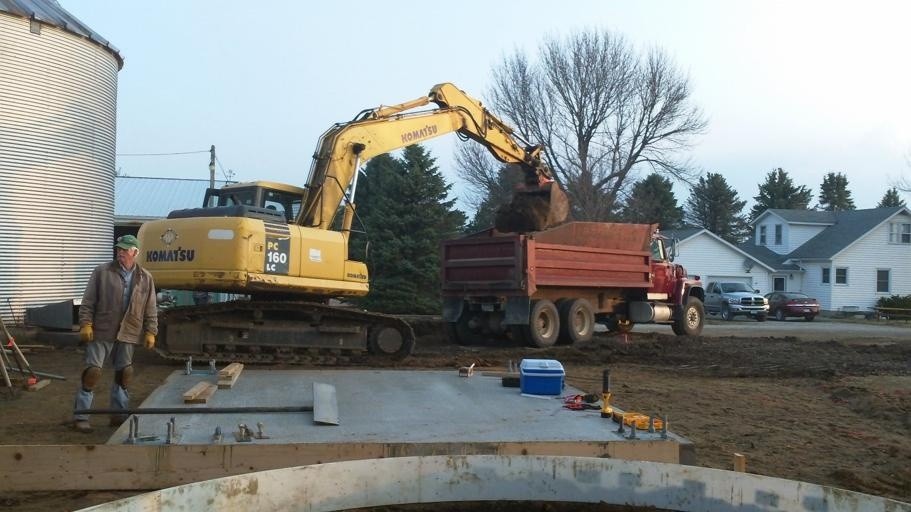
[{"x1": 519, "y1": 356, "x2": 566, "y2": 396}]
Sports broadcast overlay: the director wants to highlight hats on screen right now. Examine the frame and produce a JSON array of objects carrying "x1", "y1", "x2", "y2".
[{"x1": 113, "y1": 234, "x2": 142, "y2": 251}]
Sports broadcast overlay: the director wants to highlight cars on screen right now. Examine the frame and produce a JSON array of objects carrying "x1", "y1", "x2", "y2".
[{"x1": 760, "y1": 290, "x2": 821, "y2": 322}]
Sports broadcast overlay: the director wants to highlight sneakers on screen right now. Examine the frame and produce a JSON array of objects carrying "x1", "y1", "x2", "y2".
[
  {"x1": 74, "y1": 420, "x2": 93, "y2": 433},
  {"x1": 109, "y1": 415, "x2": 129, "y2": 426}
]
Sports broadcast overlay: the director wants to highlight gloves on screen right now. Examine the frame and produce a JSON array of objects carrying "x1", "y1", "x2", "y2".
[
  {"x1": 79, "y1": 322, "x2": 94, "y2": 342},
  {"x1": 142, "y1": 331, "x2": 156, "y2": 350}
]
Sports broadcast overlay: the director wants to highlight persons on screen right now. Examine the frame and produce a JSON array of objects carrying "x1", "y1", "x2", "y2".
[{"x1": 72, "y1": 235, "x2": 159, "y2": 432}]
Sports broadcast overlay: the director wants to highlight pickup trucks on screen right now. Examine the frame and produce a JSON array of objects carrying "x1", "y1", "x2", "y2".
[{"x1": 700, "y1": 278, "x2": 772, "y2": 323}]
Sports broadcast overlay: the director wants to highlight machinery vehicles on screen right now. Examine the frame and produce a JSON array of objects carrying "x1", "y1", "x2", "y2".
[{"x1": 130, "y1": 78, "x2": 575, "y2": 372}]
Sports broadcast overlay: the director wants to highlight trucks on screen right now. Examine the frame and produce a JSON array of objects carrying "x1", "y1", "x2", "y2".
[{"x1": 435, "y1": 210, "x2": 707, "y2": 350}]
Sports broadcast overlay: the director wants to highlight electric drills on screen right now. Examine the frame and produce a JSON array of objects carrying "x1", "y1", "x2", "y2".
[{"x1": 555, "y1": 393, "x2": 599, "y2": 405}]
